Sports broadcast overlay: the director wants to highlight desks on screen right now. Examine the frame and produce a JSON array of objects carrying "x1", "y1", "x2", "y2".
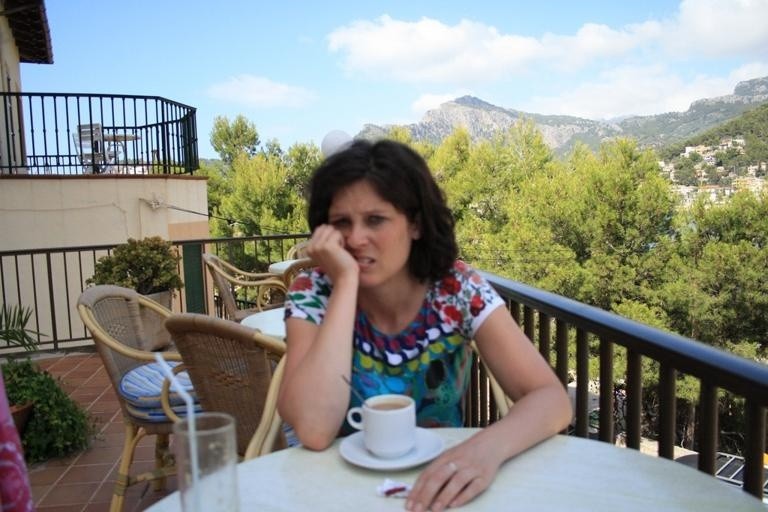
[{"x1": 149, "y1": 426, "x2": 764, "y2": 512}]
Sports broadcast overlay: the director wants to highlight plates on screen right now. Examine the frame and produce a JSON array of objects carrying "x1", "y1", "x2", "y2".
[{"x1": 339, "y1": 427, "x2": 443, "y2": 472}]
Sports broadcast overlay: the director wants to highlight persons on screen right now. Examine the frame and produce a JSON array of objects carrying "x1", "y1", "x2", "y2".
[{"x1": 276, "y1": 137, "x2": 573, "y2": 512}]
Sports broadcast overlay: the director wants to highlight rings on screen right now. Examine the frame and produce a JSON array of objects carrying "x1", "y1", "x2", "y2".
[{"x1": 446, "y1": 459, "x2": 458, "y2": 471}]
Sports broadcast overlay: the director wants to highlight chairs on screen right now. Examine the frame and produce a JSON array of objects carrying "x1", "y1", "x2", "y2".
[{"x1": 76, "y1": 237, "x2": 317, "y2": 511}]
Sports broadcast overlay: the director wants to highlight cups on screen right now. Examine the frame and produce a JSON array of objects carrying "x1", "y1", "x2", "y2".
[
  {"x1": 347, "y1": 394, "x2": 417, "y2": 461},
  {"x1": 169, "y1": 411, "x2": 240, "y2": 511}
]
